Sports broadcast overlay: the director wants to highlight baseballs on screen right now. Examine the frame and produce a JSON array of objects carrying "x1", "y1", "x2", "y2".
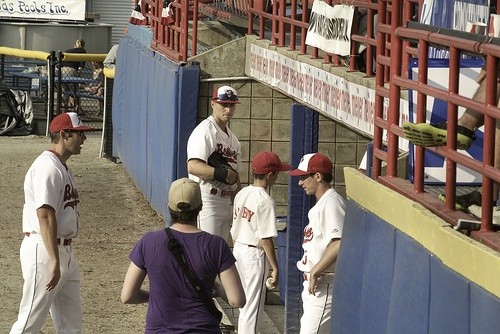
[{"x1": 266, "y1": 278, "x2": 275, "y2": 289}]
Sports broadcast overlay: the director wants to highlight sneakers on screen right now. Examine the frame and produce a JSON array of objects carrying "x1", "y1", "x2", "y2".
[{"x1": 468, "y1": 204, "x2": 500, "y2": 226}]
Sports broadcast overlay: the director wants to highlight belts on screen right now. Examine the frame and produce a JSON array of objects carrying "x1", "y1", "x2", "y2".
[
  {"x1": 211, "y1": 187, "x2": 231, "y2": 196},
  {"x1": 23, "y1": 232, "x2": 73, "y2": 247}
]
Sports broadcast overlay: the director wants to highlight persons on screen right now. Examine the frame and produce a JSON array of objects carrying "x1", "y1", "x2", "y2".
[
  {"x1": 120, "y1": 179, "x2": 246, "y2": 334},
  {"x1": 188, "y1": 86, "x2": 242, "y2": 286},
  {"x1": 46, "y1": 39, "x2": 103, "y2": 116},
  {"x1": 339, "y1": 0, "x2": 500, "y2": 210},
  {"x1": 230, "y1": 151, "x2": 291, "y2": 334},
  {"x1": 289, "y1": 153, "x2": 346, "y2": 334},
  {"x1": 9, "y1": 112, "x2": 92, "y2": 334}
]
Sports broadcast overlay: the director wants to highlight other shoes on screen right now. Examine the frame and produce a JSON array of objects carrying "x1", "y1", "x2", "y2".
[
  {"x1": 438, "y1": 188, "x2": 498, "y2": 213},
  {"x1": 402, "y1": 119, "x2": 476, "y2": 150}
]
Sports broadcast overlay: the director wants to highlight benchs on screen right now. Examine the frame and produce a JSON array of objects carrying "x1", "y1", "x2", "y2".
[{"x1": 2, "y1": 75, "x2": 104, "y2": 115}]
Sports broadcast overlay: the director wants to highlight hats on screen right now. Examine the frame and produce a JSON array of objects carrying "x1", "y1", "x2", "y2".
[
  {"x1": 211, "y1": 85, "x2": 241, "y2": 104},
  {"x1": 48, "y1": 112, "x2": 95, "y2": 133},
  {"x1": 288, "y1": 152, "x2": 333, "y2": 176},
  {"x1": 251, "y1": 151, "x2": 292, "y2": 174},
  {"x1": 169, "y1": 178, "x2": 202, "y2": 212}
]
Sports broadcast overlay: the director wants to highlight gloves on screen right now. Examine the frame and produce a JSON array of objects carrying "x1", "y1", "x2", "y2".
[{"x1": 213, "y1": 166, "x2": 238, "y2": 185}]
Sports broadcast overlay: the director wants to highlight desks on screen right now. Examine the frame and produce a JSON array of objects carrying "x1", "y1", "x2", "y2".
[{"x1": 0, "y1": 59, "x2": 96, "y2": 113}]
